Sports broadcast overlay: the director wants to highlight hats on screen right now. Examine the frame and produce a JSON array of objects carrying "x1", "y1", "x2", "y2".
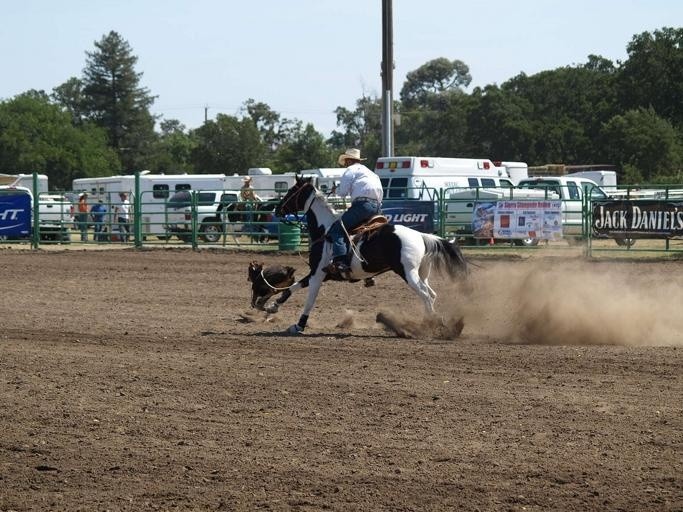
[
  {"x1": 241, "y1": 176, "x2": 253, "y2": 182},
  {"x1": 338, "y1": 148, "x2": 367, "y2": 166}
]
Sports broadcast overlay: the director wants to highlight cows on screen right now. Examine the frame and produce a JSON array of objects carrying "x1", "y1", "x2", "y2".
[{"x1": 245, "y1": 259, "x2": 296, "y2": 323}]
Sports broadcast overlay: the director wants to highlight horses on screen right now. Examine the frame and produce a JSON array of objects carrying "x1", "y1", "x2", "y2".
[
  {"x1": 215, "y1": 197, "x2": 285, "y2": 246},
  {"x1": 274, "y1": 171, "x2": 483, "y2": 334}
]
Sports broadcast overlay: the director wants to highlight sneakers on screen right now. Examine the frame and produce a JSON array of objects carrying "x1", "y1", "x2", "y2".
[{"x1": 321, "y1": 259, "x2": 349, "y2": 272}]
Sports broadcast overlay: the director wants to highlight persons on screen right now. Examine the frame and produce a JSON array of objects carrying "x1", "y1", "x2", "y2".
[
  {"x1": 113, "y1": 192, "x2": 132, "y2": 242},
  {"x1": 78, "y1": 194, "x2": 89, "y2": 241},
  {"x1": 89, "y1": 199, "x2": 107, "y2": 241},
  {"x1": 322, "y1": 148, "x2": 384, "y2": 288},
  {"x1": 241, "y1": 176, "x2": 257, "y2": 220}
]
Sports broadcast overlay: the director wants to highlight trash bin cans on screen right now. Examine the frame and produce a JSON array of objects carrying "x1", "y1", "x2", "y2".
[{"x1": 279, "y1": 221, "x2": 301, "y2": 251}]
